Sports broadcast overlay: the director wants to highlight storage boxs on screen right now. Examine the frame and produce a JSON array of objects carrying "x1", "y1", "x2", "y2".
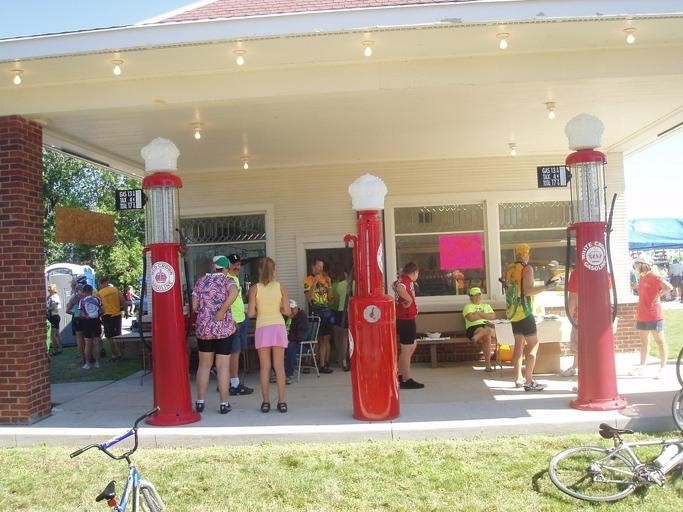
[{"x1": 521, "y1": 340, "x2": 563, "y2": 375}]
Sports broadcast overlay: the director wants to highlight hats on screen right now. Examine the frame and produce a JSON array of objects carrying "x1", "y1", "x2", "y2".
[
  {"x1": 211, "y1": 254, "x2": 240, "y2": 269},
  {"x1": 468, "y1": 286, "x2": 482, "y2": 296},
  {"x1": 634, "y1": 253, "x2": 653, "y2": 266},
  {"x1": 548, "y1": 260, "x2": 559, "y2": 268},
  {"x1": 515, "y1": 243, "x2": 531, "y2": 258}
]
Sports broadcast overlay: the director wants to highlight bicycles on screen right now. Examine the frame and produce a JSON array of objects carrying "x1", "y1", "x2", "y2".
[
  {"x1": 65, "y1": 405, "x2": 171, "y2": 512},
  {"x1": 547, "y1": 346, "x2": 683, "y2": 504}
]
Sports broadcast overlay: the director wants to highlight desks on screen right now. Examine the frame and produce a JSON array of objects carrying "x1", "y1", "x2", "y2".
[
  {"x1": 485, "y1": 311, "x2": 620, "y2": 379},
  {"x1": 112, "y1": 330, "x2": 196, "y2": 386}
]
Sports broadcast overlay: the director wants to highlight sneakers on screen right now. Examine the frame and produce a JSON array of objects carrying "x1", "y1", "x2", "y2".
[
  {"x1": 398, "y1": 376, "x2": 424, "y2": 389},
  {"x1": 468, "y1": 337, "x2": 477, "y2": 345},
  {"x1": 516, "y1": 379, "x2": 548, "y2": 392},
  {"x1": 287, "y1": 375, "x2": 295, "y2": 384},
  {"x1": 195, "y1": 400, "x2": 205, "y2": 412},
  {"x1": 220, "y1": 402, "x2": 231, "y2": 414},
  {"x1": 229, "y1": 384, "x2": 253, "y2": 395},
  {"x1": 485, "y1": 366, "x2": 495, "y2": 372},
  {"x1": 270, "y1": 373, "x2": 277, "y2": 382},
  {"x1": 82, "y1": 363, "x2": 102, "y2": 369}
]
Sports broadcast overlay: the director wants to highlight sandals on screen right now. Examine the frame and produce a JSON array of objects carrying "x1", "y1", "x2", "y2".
[
  {"x1": 303, "y1": 366, "x2": 311, "y2": 374},
  {"x1": 261, "y1": 403, "x2": 270, "y2": 413},
  {"x1": 277, "y1": 403, "x2": 287, "y2": 412},
  {"x1": 319, "y1": 368, "x2": 333, "y2": 373}
]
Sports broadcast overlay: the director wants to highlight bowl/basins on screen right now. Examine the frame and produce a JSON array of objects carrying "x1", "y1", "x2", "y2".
[{"x1": 425, "y1": 333, "x2": 441, "y2": 338}]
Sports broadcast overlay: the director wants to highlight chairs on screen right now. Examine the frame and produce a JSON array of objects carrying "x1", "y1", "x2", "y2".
[{"x1": 288, "y1": 315, "x2": 326, "y2": 383}]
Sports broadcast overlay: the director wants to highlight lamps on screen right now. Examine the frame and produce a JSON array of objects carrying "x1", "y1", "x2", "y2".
[
  {"x1": 192, "y1": 125, "x2": 202, "y2": 141},
  {"x1": 240, "y1": 155, "x2": 249, "y2": 171},
  {"x1": 507, "y1": 142, "x2": 518, "y2": 160},
  {"x1": 544, "y1": 101, "x2": 559, "y2": 122}
]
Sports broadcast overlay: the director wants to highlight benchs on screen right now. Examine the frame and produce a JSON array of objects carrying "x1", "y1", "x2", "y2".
[{"x1": 412, "y1": 334, "x2": 482, "y2": 368}]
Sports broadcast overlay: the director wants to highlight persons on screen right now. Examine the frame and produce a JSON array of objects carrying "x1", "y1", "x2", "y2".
[
  {"x1": 630, "y1": 254, "x2": 674, "y2": 375},
  {"x1": 188, "y1": 253, "x2": 242, "y2": 413},
  {"x1": 628, "y1": 256, "x2": 682, "y2": 304},
  {"x1": 389, "y1": 260, "x2": 425, "y2": 389},
  {"x1": 301, "y1": 256, "x2": 356, "y2": 373},
  {"x1": 246, "y1": 256, "x2": 291, "y2": 416},
  {"x1": 271, "y1": 295, "x2": 308, "y2": 391},
  {"x1": 504, "y1": 239, "x2": 562, "y2": 393},
  {"x1": 461, "y1": 284, "x2": 498, "y2": 377},
  {"x1": 44, "y1": 282, "x2": 63, "y2": 354},
  {"x1": 215, "y1": 252, "x2": 257, "y2": 398},
  {"x1": 66, "y1": 271, "x2": 136, "y2": 373}
]
